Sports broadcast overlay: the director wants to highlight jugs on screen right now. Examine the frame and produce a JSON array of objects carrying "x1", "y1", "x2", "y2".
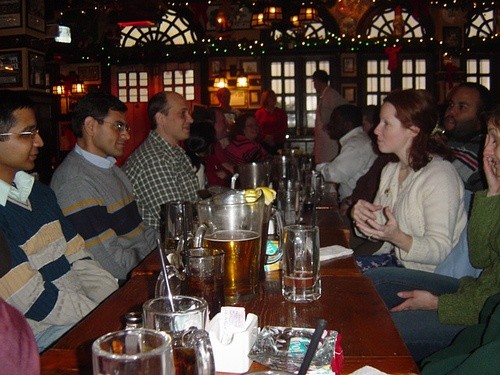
[{"x1": 194, "y1": 195, "x2": 265, "y2": 295}]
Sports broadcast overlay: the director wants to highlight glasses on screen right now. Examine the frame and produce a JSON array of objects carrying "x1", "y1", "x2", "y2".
[
  {"x1": 97, "y1": 118, "x2": 130, "y2": 132},
  {"x1": 0, "y1": 128, "x2": 39, "y2": 139}
]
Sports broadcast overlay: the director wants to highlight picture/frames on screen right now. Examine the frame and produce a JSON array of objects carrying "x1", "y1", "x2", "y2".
[
  {"x1": 0, "y1": 50, "x2": 23, "y2": 88},
  {"x1": 0, "y1": 0, "x2": 23, "y2": 29},
  {"x1": 28, "y1": 51, "x2": 46, "y2": 89},
  {"x1": 343, "y1": 86, "x2": 356, "y2": 103},
  {"x1": 210, "y1": 59, "x2": 260, "y2": 75},
  {"x1": 26, "y1": 0, "x2": 47, "y2": 33},
  {"x1": 343, "y1": 57, "x2": 355, "y2": 73},
  {"x1": 77, "y1": 65, "x2": 99, "y2": 81},
  {"x1": 249, "y1": 90, "x2": 261, "y2": 106}
]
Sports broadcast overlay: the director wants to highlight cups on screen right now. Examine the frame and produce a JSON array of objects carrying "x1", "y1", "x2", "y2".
[
  {"x1": 92, "y1": 327, "x2": 175, "y2": 375},
  {"x1": 162, "y1": 201, "x2": 195, "y2": 253},
  {"x1": 264, "y1": 205, "x2": 284, "y2": 264},
  {"x1": 141, "y1": 297, "x2": 216, "y2": 375},
  {"x1": 282, "y1": 223, "x2": 321, "y2": 302},
  {"x1": 229, "y1": 154, "x2": 319, "y2": 226},
  {"x1": 155, "y1": 247, "x2": 224, "y2": 316}
]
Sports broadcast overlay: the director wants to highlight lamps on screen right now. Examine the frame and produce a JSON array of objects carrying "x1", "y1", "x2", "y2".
[
  {"x1": 235, "y1": 60, "x2": 250, "y2": 88},
  {"x1": 52, "y1": 80, "x2": 86, "y2": 94},
  {"x1": 213, "y1": 60, "x2": 229, "y2": 88},
  {"x1": 110, "y1": 15, "x2": 162, "y2": 29},
  {"x1": 209, "y1": 6, "x2": 322, "y2": 31}
]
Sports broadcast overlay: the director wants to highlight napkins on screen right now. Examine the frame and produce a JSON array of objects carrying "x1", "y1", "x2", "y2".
[{"x1": 319, "y1": 244, "x2": 354, "y2": 260}]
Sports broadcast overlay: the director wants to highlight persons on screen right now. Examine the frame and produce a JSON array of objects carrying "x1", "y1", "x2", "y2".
[
  {"x1": 187, "y1": 88, "x2": 288, "y2": 187},
  {"x1": 311, "y1": 105, "x2": 378, "y2": 202},
  {"x1": 363, "y1": 104, "x2": 500, "y2": 367},
  {"x1": 311, "y1": 70, "x2": 348, "y2": 163},
  {"x1": 431, "y1": 82, "x2": 489, "y2": 191},
  {"x1": 0, "y1": 89, "x2": 119, "y2": 354},
  {"x1": 49, "y1": 91, "x2": 157, "y2": 283},
  {"x1": 121, "y1": 91, "x2": 200, "y2": 236},
  {"x1": 341, "y1": 155, "x2": 400, "y2": 255},
  {"x1": 350, "y1": 89, "x2": 468, "y2": 272}
]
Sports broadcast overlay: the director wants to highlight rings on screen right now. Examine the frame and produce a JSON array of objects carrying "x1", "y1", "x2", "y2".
[{"x1": 370, "y1": 234, "x2": 373, "y2": 236}]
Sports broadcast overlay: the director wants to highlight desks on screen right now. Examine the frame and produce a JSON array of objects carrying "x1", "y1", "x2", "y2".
[{"x1": 39, "y1": 183, "x2": 419, "y2": 375}]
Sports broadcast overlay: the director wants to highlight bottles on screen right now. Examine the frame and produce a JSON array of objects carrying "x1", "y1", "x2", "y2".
[{"x1": 125, "y1": 312, "x2": 142, "y2": 330}]
